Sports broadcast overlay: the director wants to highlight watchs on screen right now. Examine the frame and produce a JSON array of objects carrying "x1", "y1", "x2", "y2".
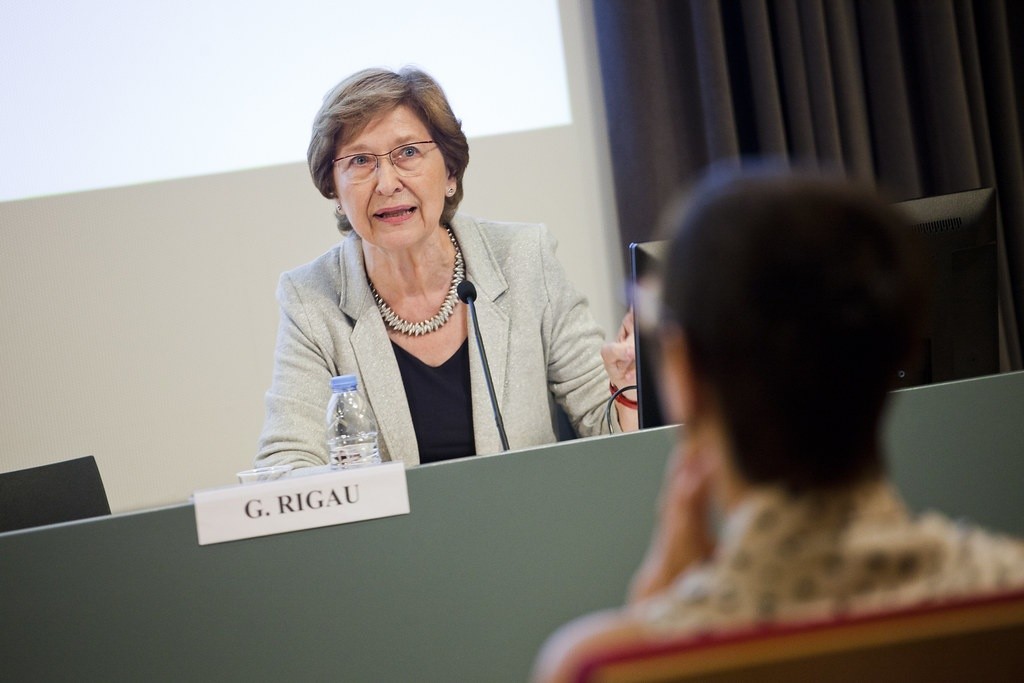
[{"x1": 608, "y1": 380, "x2": 638, "y2": 411}]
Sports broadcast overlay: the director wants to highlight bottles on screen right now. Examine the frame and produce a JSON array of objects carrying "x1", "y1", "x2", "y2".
[{"x1": 325, "y1": 374, "x2": 381, "y2": 472}]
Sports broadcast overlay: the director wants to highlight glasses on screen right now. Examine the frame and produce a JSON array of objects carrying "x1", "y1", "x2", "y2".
[{"x1": 329, "y1": 141, "x2": 438, "y2": 185}]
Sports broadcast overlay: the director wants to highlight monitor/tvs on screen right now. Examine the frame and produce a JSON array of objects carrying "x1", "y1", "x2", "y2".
[{"x1": 629, "y1": 184, "x2": 1002, "y2": 429}]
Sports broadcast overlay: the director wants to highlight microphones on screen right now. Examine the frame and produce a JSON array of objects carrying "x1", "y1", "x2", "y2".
[{"x1": 456, "y1": 280, "x2": 509, "y2": 452}]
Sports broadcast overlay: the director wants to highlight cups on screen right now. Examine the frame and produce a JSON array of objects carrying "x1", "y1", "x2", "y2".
[{"x1": 236, "y1": 465, "x2": 290, "y2": 484}]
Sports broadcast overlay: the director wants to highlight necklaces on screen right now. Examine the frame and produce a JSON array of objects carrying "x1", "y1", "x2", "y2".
[{"x1": 367, "y1": 224, "x2": 465, "y2": 336}]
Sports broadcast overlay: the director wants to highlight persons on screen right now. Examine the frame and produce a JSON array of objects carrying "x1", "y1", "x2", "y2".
[
  {"x1": 255, "y1": 67, "x2": 638, "y2": 477},
  {"x1": 531, "y1": 164, "x2": 1024, "y2": 683}
]
